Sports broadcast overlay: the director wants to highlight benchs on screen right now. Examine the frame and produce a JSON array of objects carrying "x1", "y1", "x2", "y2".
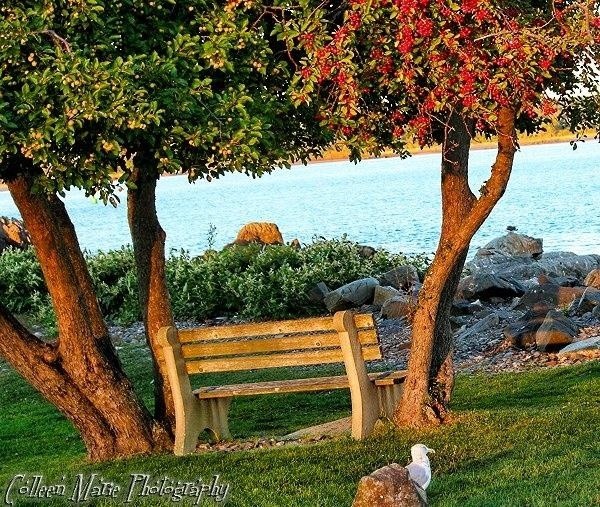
[{"x1": 155, "y1": 311, "x2": 408, "y2": 457}]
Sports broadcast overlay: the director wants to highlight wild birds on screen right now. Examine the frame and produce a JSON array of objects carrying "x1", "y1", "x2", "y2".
[
  {"x1": 506, "y1": 225, "x2": 518, "y2": 232},
  {"x1": 405, "y1": 444, "x2": 436, "y2": 504}
]
